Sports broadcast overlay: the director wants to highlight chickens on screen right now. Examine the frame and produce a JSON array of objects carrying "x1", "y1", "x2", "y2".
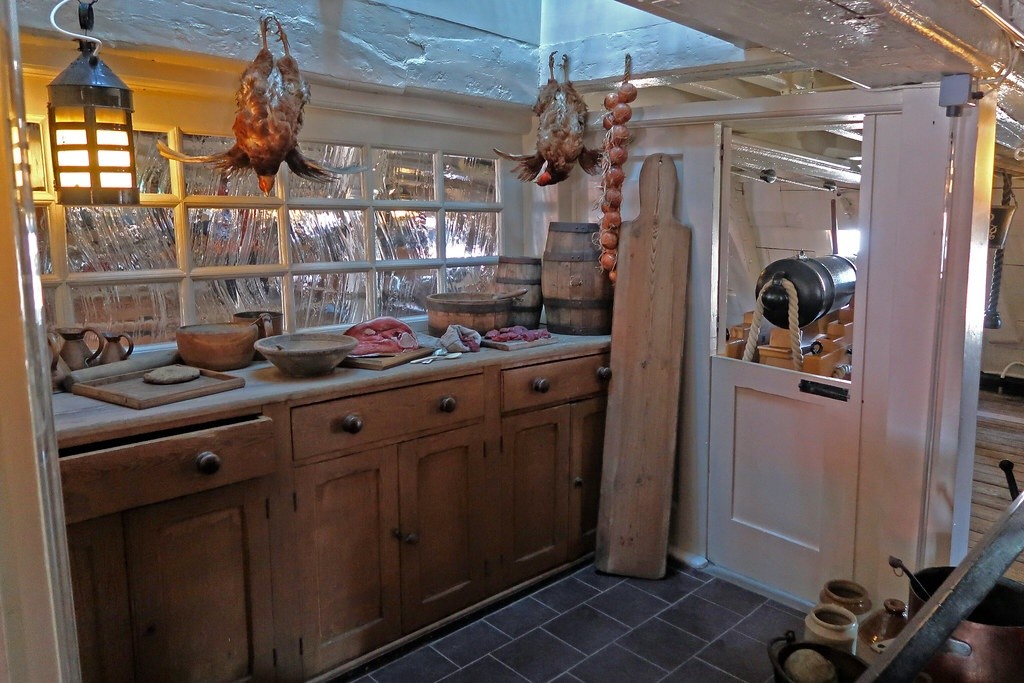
[
  {"x1": 157, "y1": 15, "x2": 370, "y2": 197},
  {"x1": 493, "y1": 51, "x2": 604, "y2": 188}
]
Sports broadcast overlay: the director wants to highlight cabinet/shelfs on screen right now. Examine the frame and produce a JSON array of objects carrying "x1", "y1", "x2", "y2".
[
  {"x1": 493, "y1": 346, "x2": 613, "y2": 597},
  {"x1": 284, "y1": 359, "x2": 493, "y2": 683},
  {"x1": 56, "y1": 399, "x2": 290, "y2": 682}
]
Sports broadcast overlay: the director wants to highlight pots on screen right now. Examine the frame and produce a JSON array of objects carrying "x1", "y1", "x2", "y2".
[
  {"x1": 767, "y1": 630, "x2": 870, "y2": 683},
  {"x1": 908, "y1": 566, "x2": 1024, "y2": 683}
]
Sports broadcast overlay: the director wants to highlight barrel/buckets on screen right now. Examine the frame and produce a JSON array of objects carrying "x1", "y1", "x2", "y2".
[
  {"x1": 495, "y1": 256, "x2": 543, "y2": 331},
  {"x1": 542, "y1": 221, "x2": 614, "y2": 335}
]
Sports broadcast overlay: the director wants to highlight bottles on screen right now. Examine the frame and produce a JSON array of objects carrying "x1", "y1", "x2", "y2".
[
  {"x1": 804, "y1": 604, "x2": 859, "y2": 655},
  {"x1": 818, "y1": 580, "x2": 872, "y2": 626},
  {"x1": 857, "y1": 599, "x2": 906, "y2": 654}
]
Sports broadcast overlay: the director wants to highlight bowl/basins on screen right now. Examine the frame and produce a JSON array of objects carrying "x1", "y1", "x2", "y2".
[
  {"x1": 426, "y1": 293, "x2": 513, "y2": 338},
  {"x1": 233, "y1": 311, "x2": 283, "y2": 361},
  {"x1": 253, "y1": 332, "x2": 359, "y2": 378},
  {"x1": 176, "y1": 323, "x2": 258, "y2": 371}
]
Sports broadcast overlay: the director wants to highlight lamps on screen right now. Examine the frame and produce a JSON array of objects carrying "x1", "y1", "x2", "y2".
[{"x1": 46, "y1": 1, "x2": 141, "y2": 207}]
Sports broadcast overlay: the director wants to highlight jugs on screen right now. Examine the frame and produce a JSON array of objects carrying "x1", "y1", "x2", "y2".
[
  {"x1": 98, "y1": 332, "x2": 134, "y2": 365},
  {"x1": 55, "y1": 327, "x2": 104, "y2": 372}
]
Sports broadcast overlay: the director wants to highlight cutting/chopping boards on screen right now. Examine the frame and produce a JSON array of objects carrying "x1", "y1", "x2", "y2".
[{"x1": 344, "y1": 345, "x2": 434, "y2": 370}]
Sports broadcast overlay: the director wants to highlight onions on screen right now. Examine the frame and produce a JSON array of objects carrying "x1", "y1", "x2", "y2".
[{"x1": 600, "y1": 84, "x2": 637, "y2": 284}]
